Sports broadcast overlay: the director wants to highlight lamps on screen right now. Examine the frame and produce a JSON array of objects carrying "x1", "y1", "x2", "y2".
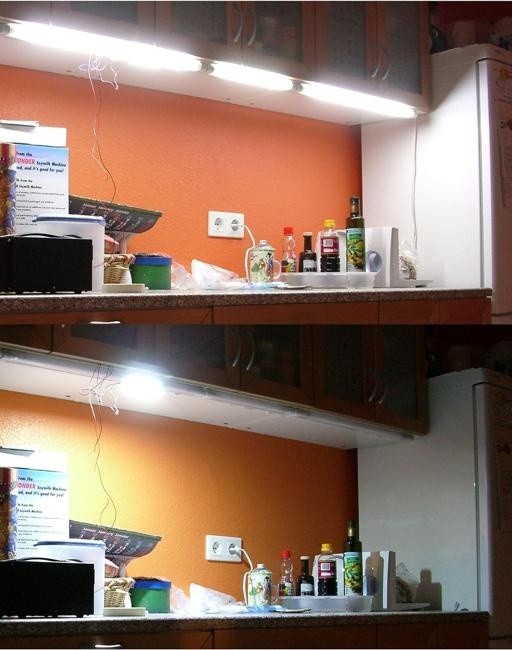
[
  {"x1": 205, "y1": 59, "x2": 298, "y2": 93},
  {"x1": 0, "y1": 18, "x2": 206, "y2": 79},
  {"x1": 295, "y1": 79, "x2": 422, "y2": 123}
]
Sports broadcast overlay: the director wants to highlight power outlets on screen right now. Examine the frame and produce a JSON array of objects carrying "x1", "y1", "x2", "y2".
[
  {"x1": 207, "y1": 210, "x2": 246, "y2": 239},
  {"x1": 204, "y1": 535, "x2": 244, "y2": 565}
]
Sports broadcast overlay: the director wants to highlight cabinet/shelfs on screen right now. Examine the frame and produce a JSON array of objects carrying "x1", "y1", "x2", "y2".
[
  {"x1": 143, "y1": 0, "x2": 316, "y2": 116},
  {"x1": 249, "y1": 1, "x2": 436, "y2": 127},
  {"x1": 240, "y1": 323, "x2": 434, "y2": 451},
  {"x1": 0, "y1": 618, "x2": 493, "y2": 650},
  {"x1": 0, "y1": 324, "x2": 158, "y2": 418},
  {"x1": 0, "y1": 2, "x2": 157, "y2": 91},
  {"x1": 0, "y1": 296, "x2": 495, "y2": 327},
  {"x1": 148, "y1": 323, "x2": 310, "y2": 432}
]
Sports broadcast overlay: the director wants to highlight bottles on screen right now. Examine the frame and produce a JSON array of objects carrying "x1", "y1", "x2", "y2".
[
  {"x1": 298, "y1": 231, "x2": 318, "y2": 273},
  {"x1": 250, "y1": 240, "x2": 274, "y2": 283},
  {"x1": 279, "y1": 543, "x2": 338, "y2": 597},
  {"x1": 345, "y1": 195, "x2": 365, "y2": 272},
  {"x1": 280, "y1": 226, "x2": 297, "y2": 272},
  {"x1": 248, "y1": 563, "x2": 273, "y2": 607},
  {"x1": 320, "y1": 220, "x2": 341, "y2": 273},
  {"x1": 343, "y1": 520, "x2": 365, "y2": 595}
]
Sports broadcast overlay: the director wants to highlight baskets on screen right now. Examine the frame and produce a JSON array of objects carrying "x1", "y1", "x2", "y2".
[
  {"x1": 105, "y1": 577, "x2": 135, "y2": 607},
  {"x1": 104, "y1": 253, "x2": 136, "y2": 283}
]
